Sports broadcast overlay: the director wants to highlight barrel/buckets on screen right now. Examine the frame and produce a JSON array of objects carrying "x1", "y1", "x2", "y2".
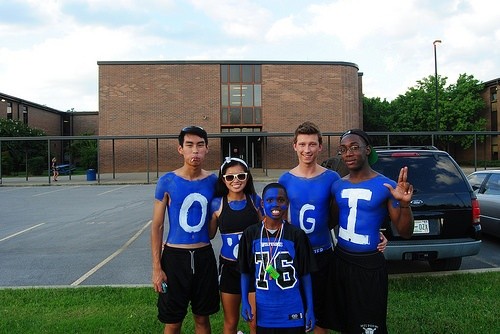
[{"x1": 87, "y1": 169, "x2": 96, "y2": 181}]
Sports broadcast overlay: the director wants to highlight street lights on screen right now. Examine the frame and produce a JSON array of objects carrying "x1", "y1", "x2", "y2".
[{"x1": 432, "y1": 39, "x2": 443, "y2": 129}]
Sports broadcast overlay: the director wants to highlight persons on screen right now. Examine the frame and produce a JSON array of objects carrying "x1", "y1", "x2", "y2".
[
  {"x1": 238, "y1": 182, "x2": 315, "y2": 334},
  {"x1": 278, "y1": 120, "x2": 388, "y2": 334},
  {"x1": 328, "y1": 128, "x2": 415, "y2": 334},
  {"x1": 209, "y1": 157, "x2": 265, "y2": 334},
  {"x1": 52, "y1": 157, "x2": 59, "y2": 181},
  {"x1": 151, "y1": 126, "x2": 220, "y2": 334}
]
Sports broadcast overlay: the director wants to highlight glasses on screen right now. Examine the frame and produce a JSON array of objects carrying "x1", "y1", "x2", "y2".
[
  {"x1": 181, "y1": 125, "x2": 205, "y2": 132},
  {"x1": 223, "y1": 173, "x2": 248, "y2": 182},
  {"x1": 337, "y1": 145, "x2": 367, "y2": 155}
]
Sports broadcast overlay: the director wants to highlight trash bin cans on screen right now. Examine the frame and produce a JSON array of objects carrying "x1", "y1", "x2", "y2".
[{"x1": 86, "y1": 169, "x2": 96, "y2": 181}]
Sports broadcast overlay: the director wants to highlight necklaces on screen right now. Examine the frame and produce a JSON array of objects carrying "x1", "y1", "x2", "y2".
[{"x1": 258, "y1": 219, "x2": 283, "y2": 281}]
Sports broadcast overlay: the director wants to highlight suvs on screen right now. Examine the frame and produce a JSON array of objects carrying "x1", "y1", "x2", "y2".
[{"x1": 320, "y1": 146, "x2": 482, "y2": 271}]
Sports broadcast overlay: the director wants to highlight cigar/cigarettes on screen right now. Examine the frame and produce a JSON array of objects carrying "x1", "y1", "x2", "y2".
[{"x1": 191, "y1": 158, "x2": 194, "y2": 163}]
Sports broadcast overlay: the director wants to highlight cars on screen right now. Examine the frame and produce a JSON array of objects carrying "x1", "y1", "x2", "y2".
[{"x1": 464, "y1": 169, "x2": 499, "y2": 242}]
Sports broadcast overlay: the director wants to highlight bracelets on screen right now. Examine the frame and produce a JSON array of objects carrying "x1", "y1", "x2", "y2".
[{"x1": 400, "y1": 206, "x2": 410, "y2": 209}]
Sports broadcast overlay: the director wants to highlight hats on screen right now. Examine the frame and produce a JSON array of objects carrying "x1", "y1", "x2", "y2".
[{"x1": 340, "y1": 129, "x2": 378, "y2": 166}]
